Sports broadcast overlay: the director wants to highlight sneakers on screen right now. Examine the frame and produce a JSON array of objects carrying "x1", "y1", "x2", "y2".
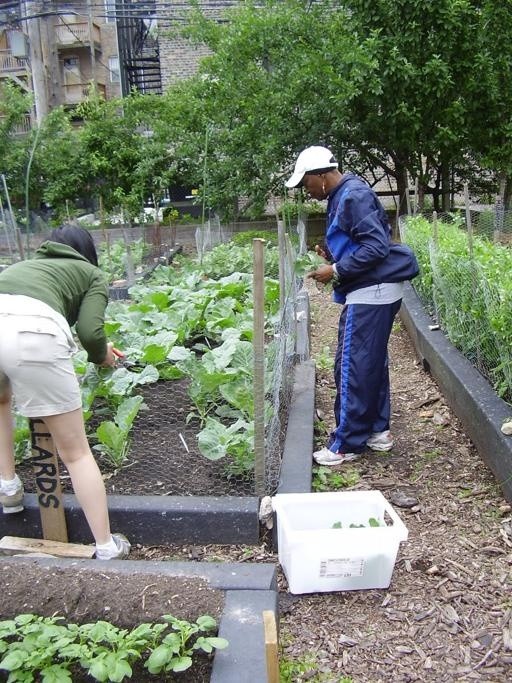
[
  {"x1": 94, "y1": 531, "x2": 132, "y2": 560},
  {"x1": 367, "y1": 430, "x2": 394, "y2": 452},
  {"x1": 0, "y1": 484, "x2": 26, "y2": 514},
  {"x1": 312, "y1": 446, "x2": 361, "y2": 466}
]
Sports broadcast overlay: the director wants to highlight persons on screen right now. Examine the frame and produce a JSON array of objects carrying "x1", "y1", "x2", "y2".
[
  {"x1": 286, "y1": 141, "x2": 420, "y2": 467},
  {"x1": 1, "y1": 219, "x2": 132, "y2": 564}
]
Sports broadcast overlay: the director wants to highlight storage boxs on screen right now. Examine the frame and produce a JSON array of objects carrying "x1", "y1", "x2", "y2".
[{"x1": 274, "y1": 489, "x2": 409, "y2": 595}]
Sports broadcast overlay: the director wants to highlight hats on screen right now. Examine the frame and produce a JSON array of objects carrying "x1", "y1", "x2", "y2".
[{"x1": 284, "y1": 144, "x2": 339, "y2": 188}]
